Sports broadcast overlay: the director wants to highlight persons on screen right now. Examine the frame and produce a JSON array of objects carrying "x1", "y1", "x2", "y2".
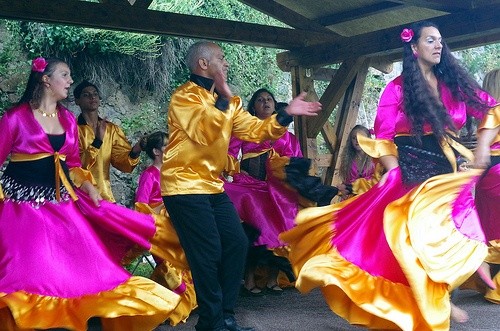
[
  {"x1": 275, "y1": 19, "x2": 499, "y2": 331},
  {"x1": 161, "y1": 40, "x2": 322, "y2": 331}
]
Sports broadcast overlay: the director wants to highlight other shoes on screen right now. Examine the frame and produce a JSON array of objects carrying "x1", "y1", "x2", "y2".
[
  {"x1": 450, "y1": 305, "x2": 468, "y2": 323},
  {"x1": 266, "y1": 285, "x2": 284, "y2": 294},
  {"x1": 244, "y1": 285, "x2": 263, "y2": 295},
  {"x1": 477, "y1": 268, "x2": 495, "y2": 289}
]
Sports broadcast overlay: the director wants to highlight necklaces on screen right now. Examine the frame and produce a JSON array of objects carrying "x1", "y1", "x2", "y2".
[
  {"x1": 0, "y1": 56, "x2": 500, "y2": 331},
  {"x1": 36, "y1": 106, "x2": 59, "y2": 118}
]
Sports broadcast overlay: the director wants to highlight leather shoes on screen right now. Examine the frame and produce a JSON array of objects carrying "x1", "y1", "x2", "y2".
[{"x1": 224, "y1": 316, "x2": 255, "y2": 331}]
